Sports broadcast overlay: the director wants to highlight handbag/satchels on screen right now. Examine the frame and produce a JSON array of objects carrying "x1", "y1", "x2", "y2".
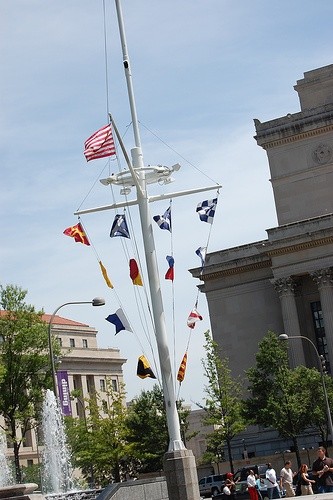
[{"x1": 223, "y1": 486, "x2": 231, "y2": 495}]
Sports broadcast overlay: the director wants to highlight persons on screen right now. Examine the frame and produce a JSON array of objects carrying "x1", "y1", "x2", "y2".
[{"x1": 222, "y1": 446, "x2": 333, "y2": 500}]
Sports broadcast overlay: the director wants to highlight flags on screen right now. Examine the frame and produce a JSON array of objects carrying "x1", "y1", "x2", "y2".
[
  {"x1": 136, "y1": 355, "x2": 156, "y2": 381},
  {"x1": 109, "y1": 213, "x2": 131, "y2": 239},
  {"x1": 186, "y1": 303, "x2": 204, "y2": 329},
  {"x1": 193, "y1": 196, "x2": 219, "y2": 224},
  {"x1": 99, "y1": 261, "x2": 115, "y2": 289},
  {"x1": 129, "y1": 258, "x2": 143, "y2": 287},
  {"x1": 164, "y1": 255, "x2": 175, "y2": 283},
  {"x1": 104, "y1": 308, "x2": 133, "y2": 336},
  {"x1": 152, "y1": 207, "x2": 173, "y2": 234},
  {"x1": 83, "y1": 124, "x2": 118, "y2": 163},
  {"x1": 176, "y1": 352, "x2": 188, "y2": 383},
  {"x1": 62, "y1": 223, "x2": 91, "y2": 246},
  {"x1": 195, "y1": 246, "x2": 207, "y2": 277}
]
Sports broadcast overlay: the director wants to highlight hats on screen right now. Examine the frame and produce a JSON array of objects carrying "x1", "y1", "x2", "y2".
[{"x1": 227, "y1": 473, "x2": 234, "y2": 478}]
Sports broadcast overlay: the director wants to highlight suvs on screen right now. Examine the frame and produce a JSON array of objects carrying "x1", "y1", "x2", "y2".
[{"x1": 198, "y1": 463, "x2": 270, "y2": 500}]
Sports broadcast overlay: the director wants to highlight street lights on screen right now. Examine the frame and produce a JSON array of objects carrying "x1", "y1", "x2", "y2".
[
  {"x1": 48, "y1": 297, "x2": 106, "y2": 492},
  {"x1": 274, "y1": 449, "x2": 291, "y2": 466},
  {"x1": 302, "y1": 447, "x2": 314, "y2": 469},
  {"x1": 70, "y1": 396, "x2": 95, "y2": 489},
  {"x1": 279, "y1": 334, "x2": 333, "y2": 442},
  {"x1": 216, "y1": 451, "x2": 222, "y2": 475}
]
